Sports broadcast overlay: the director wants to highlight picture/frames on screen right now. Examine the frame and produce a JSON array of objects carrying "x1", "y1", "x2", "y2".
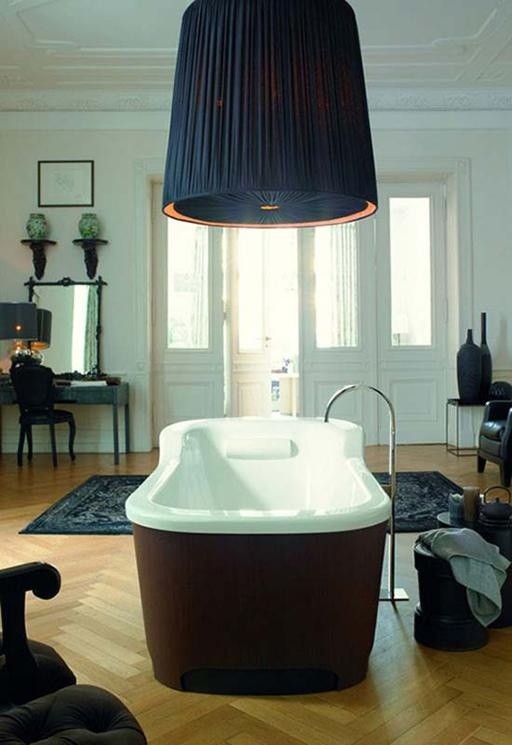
[{"x1": 35, "y1": 158, "x2": 97, "y2": 210}]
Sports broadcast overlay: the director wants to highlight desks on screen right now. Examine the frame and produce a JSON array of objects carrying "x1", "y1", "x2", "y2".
[
  {"x1": 434, "y1": 506, "x2": 511, "y2": 632},
  {"x1": 0, "y1": 376, "x2": 134, "y2": 474},
  {"x1": 444, "y1": 396, "x2": 511, "y2": 458}
]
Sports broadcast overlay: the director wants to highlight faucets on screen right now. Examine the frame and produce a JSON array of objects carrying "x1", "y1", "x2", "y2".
[{"x1": 321, "y1": 384, "x2": 411, "y2": 602}]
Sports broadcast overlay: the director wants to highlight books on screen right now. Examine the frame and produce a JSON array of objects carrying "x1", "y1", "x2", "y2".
[{"x1": 56, "y1": 380, "x2": 109, "y2": 389}]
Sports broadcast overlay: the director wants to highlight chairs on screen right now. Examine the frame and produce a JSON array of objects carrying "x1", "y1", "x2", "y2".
[
  {"x1": 1, "y1": 557, "x2": 152, "y2": 745},
  {"x1": 7, "y1": 362, "x2": 78, "y2": 472},
  {"x1": 474, "y1": 399, "x2": 512, "y2": 490}
]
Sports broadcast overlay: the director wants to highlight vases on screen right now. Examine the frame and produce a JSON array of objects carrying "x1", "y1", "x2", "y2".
[
  {"x1": 78, "y1": 210, "x2": 102, "y2": 241},
  {"x1": 455, "y1": 326, "x2": 484, "y2": 403},
  {"x1": 476, "y1": 308, "x2": 495, "y2": 398},
  {"x1": 24, "y1": 210, "x2": 50, "y2": 242}
]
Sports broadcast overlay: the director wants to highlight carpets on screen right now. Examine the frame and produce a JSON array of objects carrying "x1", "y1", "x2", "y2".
[{"x1": 14, "y1": 469, "x2": 489, "y2": 535}]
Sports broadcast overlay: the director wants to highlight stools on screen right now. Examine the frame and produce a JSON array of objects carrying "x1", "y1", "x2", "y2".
[{"x1": 409, "y1": 538, "x2": 495, "y2": 655}]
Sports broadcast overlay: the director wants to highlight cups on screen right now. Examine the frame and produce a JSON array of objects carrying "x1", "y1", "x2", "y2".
[
  {"x1": 463, "y1": 486, "x2": 480, "y2": 523},
  {"x1": 448, "y1": 493, "x2": 465, "y2": 519}
]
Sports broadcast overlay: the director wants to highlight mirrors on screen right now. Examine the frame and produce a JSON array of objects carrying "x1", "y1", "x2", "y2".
[{"x1": 24, "y1": 275, "x2": 113, "y2": 380}]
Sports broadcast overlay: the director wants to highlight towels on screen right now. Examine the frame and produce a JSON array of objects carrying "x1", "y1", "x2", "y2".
[{"x1": 412, "y1": 527, "x2": 510, "y2": 628}]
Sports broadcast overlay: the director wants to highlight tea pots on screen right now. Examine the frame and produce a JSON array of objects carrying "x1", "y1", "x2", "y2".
[{"x1": 479, "y1": 484, "x2": 512, "y2": 529}]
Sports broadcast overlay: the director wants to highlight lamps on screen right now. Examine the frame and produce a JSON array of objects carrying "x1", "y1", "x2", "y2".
[
  {"x1": 157, "y1": 0, "x2": 382, "y2": 233},
  {"x1": 0, "y1": 299, "x2": 40, "y2": 376},
  {"x1": 28, "y1": 306, "x2": 56, "y2": 364}
]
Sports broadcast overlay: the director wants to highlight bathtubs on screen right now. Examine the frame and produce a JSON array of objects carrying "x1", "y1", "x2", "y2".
[{"x1": 125, "y1": 417, "x2": 391, "y2": 536}]
